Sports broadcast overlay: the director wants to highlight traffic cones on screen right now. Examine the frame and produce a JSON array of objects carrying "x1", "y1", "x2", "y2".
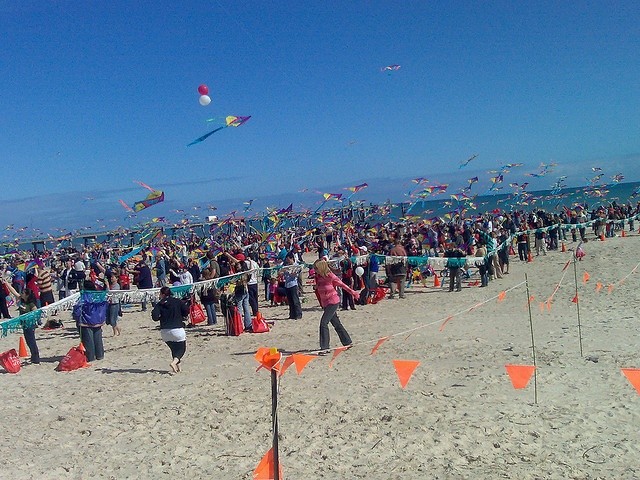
[
  {"x1": 601, "y1": 233, "x2": 605, "y2": 241},
  {"x1": 562, "y1": 242, "x2": 566, "y2": 251},
  {"x1": 434, "y1": 273, "x2": 439, "y2": 286},
  {"x1": 622, "y1": 230, "x2": 626, "y2": 236},
  {"x1": 78, "y1": 343, "x2": 88, "y2": 368},
  {"x1": 18, "y1": 336, "x2": 28, "y2": 357},
  {"x1": 527, "y1": 252, "x2": 532, "y2": 262}
]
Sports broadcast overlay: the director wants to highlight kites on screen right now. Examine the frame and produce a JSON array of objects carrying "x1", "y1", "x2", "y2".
[
  {"x1": 381, "y1": 64, "x2": 402, "y2": 79},
  {"x1": 185, "y1": 114, "x2": 254, "y2": 149},
  {"x1": 1, "y1": 154, "x2": 639, "y2": 251}
]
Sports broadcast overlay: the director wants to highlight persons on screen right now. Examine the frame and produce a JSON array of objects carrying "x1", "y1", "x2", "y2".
[{"x1": 1, "y1": 199, "x2": 640, "y2": 377}]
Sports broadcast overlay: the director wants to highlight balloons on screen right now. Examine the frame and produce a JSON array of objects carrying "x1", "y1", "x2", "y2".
[
  {"x1": 199, "y1": 95, "x2": 212, "y2": 106},
  {"x1": 198, "y1": 84, "x2": 208, "y2": 95}
]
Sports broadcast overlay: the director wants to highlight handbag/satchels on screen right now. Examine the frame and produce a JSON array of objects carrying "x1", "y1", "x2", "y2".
[
  {"x1": 0, "y1": 349, "x2": 21, "y2": 373},
  {"x1": 55, "y1": 347, "x2": 88, "y2": 371},
  {"x1": 252, "y1": 317, "x2": 270, "y2": 333},
  {"x1": 189, "y1": 303, "x2": 206, "y2": 325},
  {"x1": 235, "y1": 284, "x2": 247, "y2": 296},
  {"x1": 48, "y1": 320, "x2": 63, "y2": 329}
]
